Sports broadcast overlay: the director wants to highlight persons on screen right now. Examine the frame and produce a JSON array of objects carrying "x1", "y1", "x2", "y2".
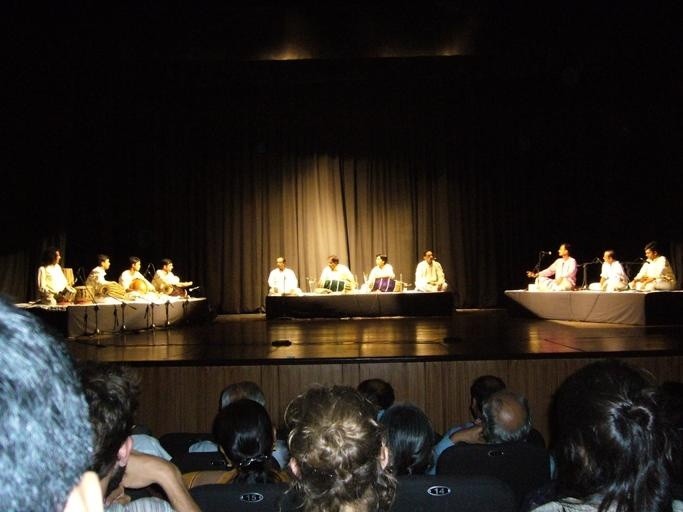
[
  {"x1": 527, "y1": 244, "x2": 577, "y2": 291},
  {"x1": 266, "y1": 255, "x2": 303, "y2": 298},
  {"x1": 37, "y1": 244, "x2": 71, "y2": 315},
  {"x1": 425, "y1": 390, "x2": 533, "y2": 474},
  {"x1": 531, "y1": 361, "x2": 681, "y2": 510},
  {"x1": 120, "y1": 363, "x2": 173, "y2": 461},
  {"x1": 73, "y1": 363, "x2": 201, "y2": 512},
  {"x1": 274, "y1": 384, "x2": 396, "y2": 510},
  {"x1": 83, "y1": 253, "x2": 111, "y2": 299},
  {"x1": 182, "y1": 400, "x2": 289, "y2": 490},
  {"x1": 318, "y1": 255, "x2": 354, "y2": 291},
  {"x1": 471, "y1": 375, "x2": 545, "y2": 448},
  {"x1": 188, "y1": 381, "x2": 288, "y2": 454},
  {"x1": 588, "y1": 251, "x2": 629, "y2": 291},
  {"x1": 413, "y1": 249, "x2": 448, "y2": 292},
  {"x1": 118, "y1": 258, "x2": 151, "y2": 299},
  {"x1": 379, "y1": 404, "x2": 433, "y2": 475},
  {"x1": 631, "y1": 242, "x2": 677, "y2": 291},
  {"x1": 154, "y1": 257, "x2": 182, "y2": 299},
  {"x1": 0, "y1": 291, "x2": 103, "y2": 512},
  {"x1": 357, "y1": 378, "x2": 395, "y2": 409},
  {"x1": 360, "y1": 252, "x2": 394, "y2": 291}
]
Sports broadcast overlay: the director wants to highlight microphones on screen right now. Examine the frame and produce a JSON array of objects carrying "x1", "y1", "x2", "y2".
[
  {"x1": 596, "y1": 258, "x2": 603, "y2": 265},
  {"x1": 75, "y1": 267, "x2": 81, "y2": 277},
  {"x1": 282, "y1": 275, "x2": 287, "y2": 295},
  {"x1": 640, "y1": 257, "x2": 650, "y2": 264},
  {"x1": 541, "y1": 250, "x2": 552, "y2": 256},
  {"x1": 144, "y1": 265, "x2": 151, "y2": 276}
]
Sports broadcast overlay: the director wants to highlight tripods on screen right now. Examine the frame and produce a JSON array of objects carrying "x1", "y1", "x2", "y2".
[
  {"x1": 144, "y1": 303, "x2": 169, "y2": 336},
  {"x1": 116, "y1": 303, "x2": 142, "y2": 335},
  {"x1": 88, "y1": 304, "x2": 114, "y2": 338}
]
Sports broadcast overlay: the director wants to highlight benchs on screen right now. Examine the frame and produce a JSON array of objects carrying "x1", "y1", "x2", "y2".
[
  {"x1": 504, "y1": 289, "x2": 681, "y2": 324},
  {"x1": 265, "y1": 293, "x2": 456, "y2": 317},
  {"x1": 15, "y1": 297, "x2": 208, "y2": 337}
]
